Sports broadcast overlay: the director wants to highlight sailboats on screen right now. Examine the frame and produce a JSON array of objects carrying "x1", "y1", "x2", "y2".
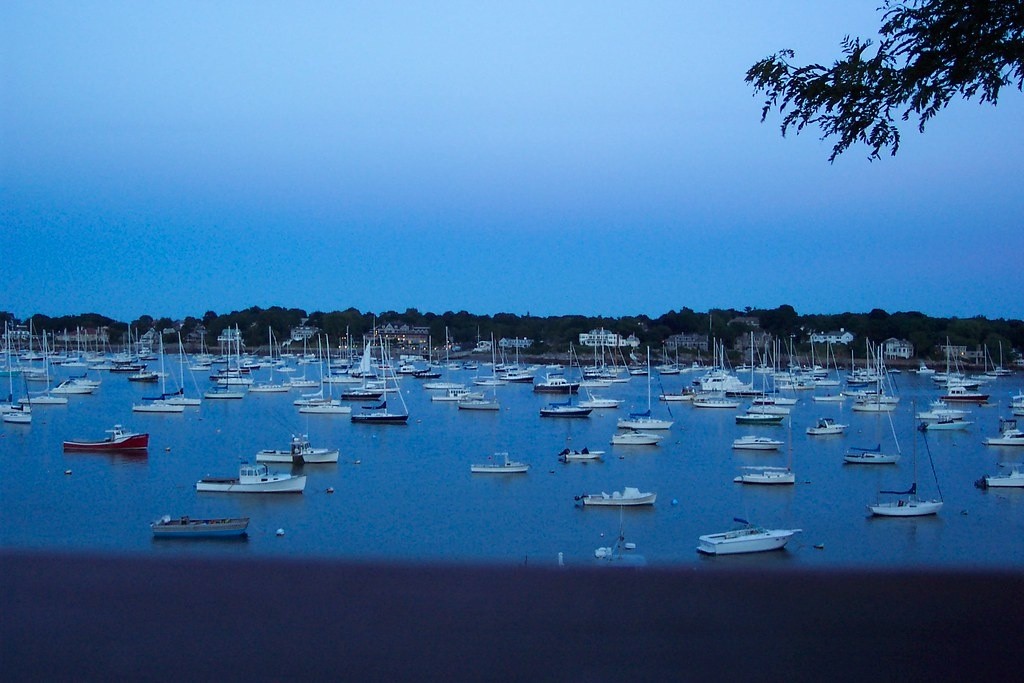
[
  {"x1": 655, "y1": 337, "x2": 719, "y2": 374},
  {"x1": 0, "y1": 317, "x2": 167, "y2": 383},
  {"x1": 424, "y1": 327, "x2": 469, "y2": 389},
  {"x1": 865, "y1": 399, "x2": 944, "y2": 516},
  {"x1": 293, "y1": 332, "x2": 353, "y2": 413},
  {"x1": 132, "y1": 331, "x2": 186, "y2": 413},
  {"x1": 350, "y1": 335, "x2": 409, "y2": 424},
  {"x1": 735, "y1": 332, "x2": 885, "y2": 389},
  {"x1": 18, "y1": 334, "x2": 69, "y2": 404},
  {"x1": 0, "y1": 328, "x2": 33, "y2": 423},
  {"x1": 248, "y1": 326, "x2": 293, "y2": 392},
  {"x1": 736, "y1": 374, "x2": 790, "y2": 426},
  {"x1": 444, "y1": 326, "x2": 526, "y2": 372},
  {"x1": 177, "y1": 322, "x2": 442, "y2": 378},
  {"x1": 539, "y1": 341, "x2": 593, "y2": 418},
  {"x1": 617, "y1": 346, "x2": 674, "y2": 430},
  {"x1": 577, "y1": 327, "x2": 648, "y2": 386},
  {"x1": 842, "y1": 366, "x2": 902, "y2": 464},
  {"x1": 572, "y1": 346, "x2": 625, "y2": 408},
  {"x1": 983, "y1": 340, "x2": 1016, "y2": 376},
  {"x1": 203, "y1": 325, "x2": 247, "y2": 398},
  {"x1": 154, "y1": 330, "x2": 203, "y2": 406},
  {"x1": 841, "y1": 343, "x2": 899, "y2": 412},
  {"x1": 693, "y1": 336, "x2": 754, "y2": 392},
  {"x1": 930, "y1": 335, "x2": 965, "y2": 381}
]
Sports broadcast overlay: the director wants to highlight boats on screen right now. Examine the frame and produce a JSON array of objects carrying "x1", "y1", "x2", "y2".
[
  {"x1": 64, "y1": 424, "x2": 149, "y2": 449},
  {"x1": 919, "y1": 414, "x2": 973, "y2": 429},
  {"x1": 731, "y1": 436, "x2": 785, "y2": 450},
  {"x1": 533, "y1": 371, "x2": 580, "y2": 393},
  {"x1": 471, "y1": 452, "x2": 531, "y2": 473},
  {"x1": 691, "y1": 389, "x2": 741, "y2": 408},
  {"x1": 982, "y1": 417, "x2": 1024, "y2": 445},
  {"x1": 583, "y1": 487, "x2": 657, "y2": 506},
  {"x1": 565, "y1": 448, "x2": 604, "y2": 460},
  {"x1": 915, "y1": 400, "x2": 971, "y2": 420},
  {"x1": 805, "y1": 417, "x2": 848, "y2": 434},
  {"x1": 696, "y1": 528, "x2": 804, "y2": 554},
  {"x1": 888, "y1": 367, "x2": 901, "y2": 373},
  {"x1": 527, "y1": 362, "x2": 563, "y2": 372},
  {"x1": 985, "y1": 469, "x2": 1024, "y2": 487},
  {"x1": 432, "y1": 389, "x2": 484, "y2": 400},
  {"x1": 196, "y1": 461, "x2": 307, "y2": 493},
  {"x1": 1008, "y1": 388, "x2": 1024, "y2": 417},
  {"x1": 610, "y1": 429, "x2": 663, "y2": 445},
  {"x1": 256, "y1": 416, "x2": 339, "y2": 463},
  {"x1": 455, "y1": 393, "x2": 500, "y2": 410},
  {"x1": 150, "y1": 516, "x2": 249, "y2": 536},
  {"x1": 733, "y1": 472, "x2": 796, "y2": 485},
  {"x1": 940, "y1": 376, "x2": 984, "y2": 389},
  {"x1": 660, "y1": 384, "x2": 698, "y2": 401},
  {"x1": 916, "y1": 364, "x2": 936, "y2": 374},
  {"x1": 941, "y1": 387, "x2": 990, "y2": 403},
  {"x1": 813, "y1": 396, "x2": 847, "y2": 401}
]
